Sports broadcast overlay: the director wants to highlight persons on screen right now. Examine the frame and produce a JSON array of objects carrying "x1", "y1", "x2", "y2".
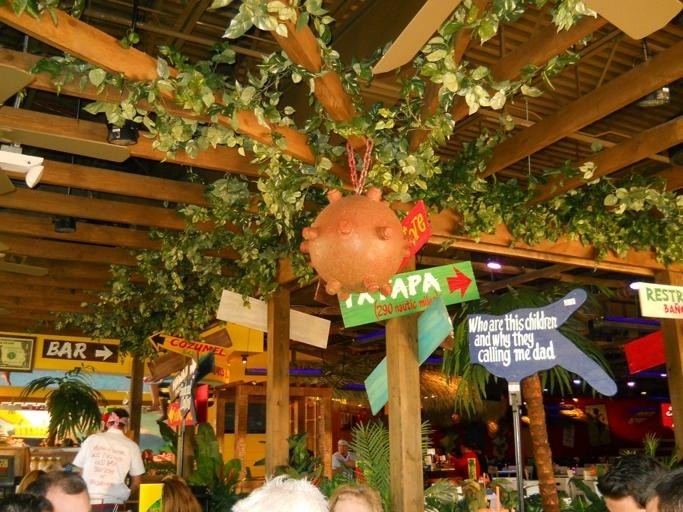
[
  {"x1": 71, "y1": 407, "x2": 146, "y2": 512},
  {"x1": 329, "y1": 482, "x2": 383, "y2": 512},
  {"x1": 596, "y1": 454, "x2": 665, "y2": 512},
  {"x1": 447, "y1": 442, "x2": 482, "y2": 480},
  {"x1": 230, "y1": 474, "x2": 328, "y2": 512},
  {"x1": 472, "y1": 442, "x2": 489, "y2": 474},
  {"x1": 0, "y1": 493, "x2": 54, "y2": 512},
  {"x1": 644, "y1": 468, "x2": 683, "y2": 512},
  {"x1": 26, "y1": 470, "x2": 92, "y2": 512},
  {"x1": 160, "y1": 474, "x2": 203, "y2": 512},
  {"x1": 332, "y1": 439, "x2": 355, "y2": 469},
  {"x1": 17, "y1": 469, "x2": 47, "y2": 495}
]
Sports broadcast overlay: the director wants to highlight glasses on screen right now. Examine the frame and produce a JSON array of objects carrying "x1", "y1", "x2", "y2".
[
  {"x1": 105, "y1": 1, "x2": 140, "y2": 146},
  {"x1": 630, "y1": 40, "x2": 669, "y2": 109},
  {"x1": 51, "y1": 97, "x2": 82, "y2": 233},
  {"x1": 630, "y1": 274, "x2": 647, "y2": 290},
  {"x1": 488, "y1": 254, "x2": 504, "y2": 271}
]
